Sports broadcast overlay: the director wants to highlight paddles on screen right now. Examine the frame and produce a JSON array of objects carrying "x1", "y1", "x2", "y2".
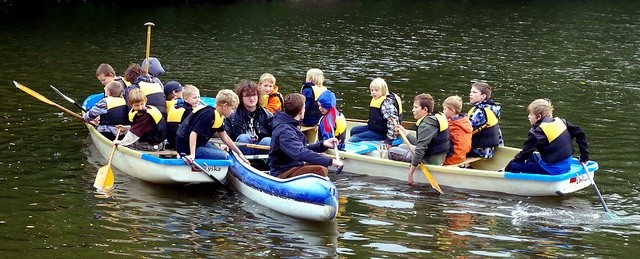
[
  {"x1": 391, "y1": 118, "x2": 444, "y2": 194},
  {"x1": 345, "y1": 117, "x2": 417, "y2": 129},
  {"x1": 93, "y1": 128, "x2": 123, "y2": 198},
  {"x1": 14, "y1": 81, "x2": 83, "y2": 121},
  {"x1": 50, "y1": 84, "x2": 87, "y2": 113}
]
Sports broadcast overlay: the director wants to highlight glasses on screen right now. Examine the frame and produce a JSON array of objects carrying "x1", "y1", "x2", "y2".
[{"x1": 242, "y1": 94, "x2": 257, "y2": 97}]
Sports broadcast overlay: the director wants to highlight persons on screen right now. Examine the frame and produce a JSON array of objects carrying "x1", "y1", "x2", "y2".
[
  {"x1": 224, "y1": 82, "x2": 274, "y2": 153},
  {"x1": 142, "y1": 56, "x2": 167, "y2": 84},
  {"x1": 93, "y1": 61, "x2": 128, "y2": 90},
  {"x1": 316, "y1": 90, "x2": 347, "y2": 151},
  {"x1": 269, "y1": 92, "x2": 342, "y2": 178},
  {"x1": 258, "y1": 73, "x2": 284, "y2": 114},
  {"x1": 299, "y1": 67, "x2": 327, "y2": 126},
  {"x1": 172, "y1": 88, "x2": 250, "y2": 169},
  {"x1": 468, "y1": 82, "x2": 502, "y2": 158},
  {"x1": 164, "y1": 84, "x2": 203, "y2": 151},
  {"x1": 348, "y1": 76, "x2": 403, "y2": 149},
  {"x1": 442, "y1": 95, "x2": 474, "y2": 165},
  {"x1": 81, "y1": 80, "x2": 128, "y2": 138},
  {"x1": 122, "y1": 64, "x2": 166, "y2": 115},
  {"x1": 502, "y1": 96, "x2": 589, "y2": 175},
  {"x1": 113, "y1": 87, "x2": 166, "y2": 152},
  {"x1": 388, "y1": 93, "x2": 451, "y2": 185},
  {"x1": 164, "y1": 80, "x2": 188, "y2": 115}
]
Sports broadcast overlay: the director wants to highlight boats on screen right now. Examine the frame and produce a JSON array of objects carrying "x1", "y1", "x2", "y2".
[
  {"x1": 296, "y1": 118, "x2": 599, "y2": 197},
  {"x1": 79, "y1": 91, "x2": 233, "y2": 186},
  {"x1": 176, "y1": 95, "x2": 339, "y2": 222}
]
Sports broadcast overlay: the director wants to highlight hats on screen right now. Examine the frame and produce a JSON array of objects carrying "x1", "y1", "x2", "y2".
[{"x1": 164, "y1": 81, "x2": 184, "y2": 96}]
